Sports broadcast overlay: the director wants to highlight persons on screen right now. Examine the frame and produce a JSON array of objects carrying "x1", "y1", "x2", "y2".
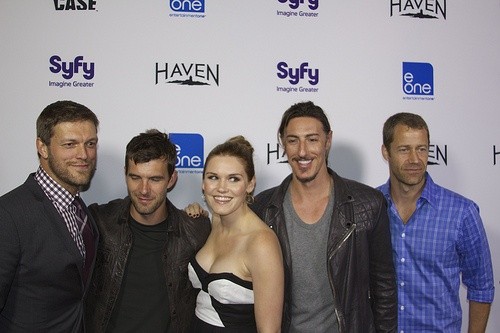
[
  {"x1": 245, "y1": 100, "x2": 400, "y2": 333},
  {"x1": 0, "y1": 101, "x2": 101, "y2": 333},
  {"x1": 180, "y1": 135, "x2": 284, "y2": 333},
  {"x1": 86, "y1": 128, "x2": 212, "y2": 333},
  {"x1": 371, "y1": 114, "x2": 494, "y2": 333}
]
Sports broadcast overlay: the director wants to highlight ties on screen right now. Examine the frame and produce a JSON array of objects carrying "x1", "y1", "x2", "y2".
[{"x1": 72, "y1": 195, "x2": 97, "y2": 286}]
